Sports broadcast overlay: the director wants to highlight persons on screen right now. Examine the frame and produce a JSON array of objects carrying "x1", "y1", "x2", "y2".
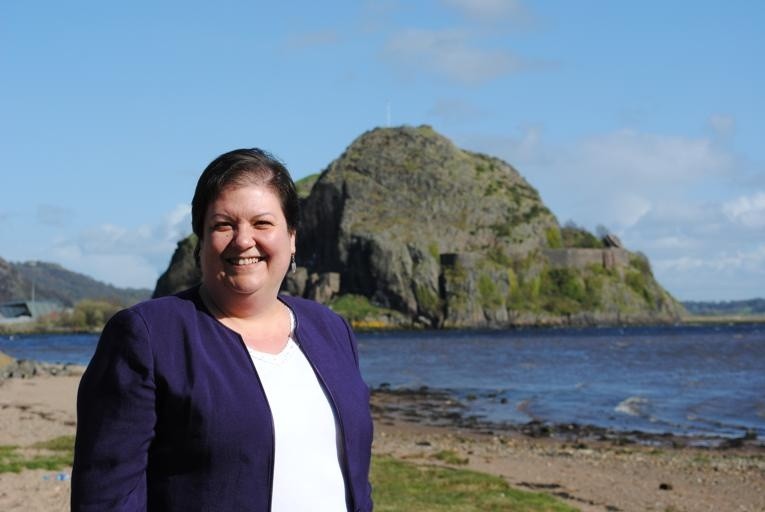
[{"x1": 70, "y1": 148, "x2": 375, "y2": 512}]
